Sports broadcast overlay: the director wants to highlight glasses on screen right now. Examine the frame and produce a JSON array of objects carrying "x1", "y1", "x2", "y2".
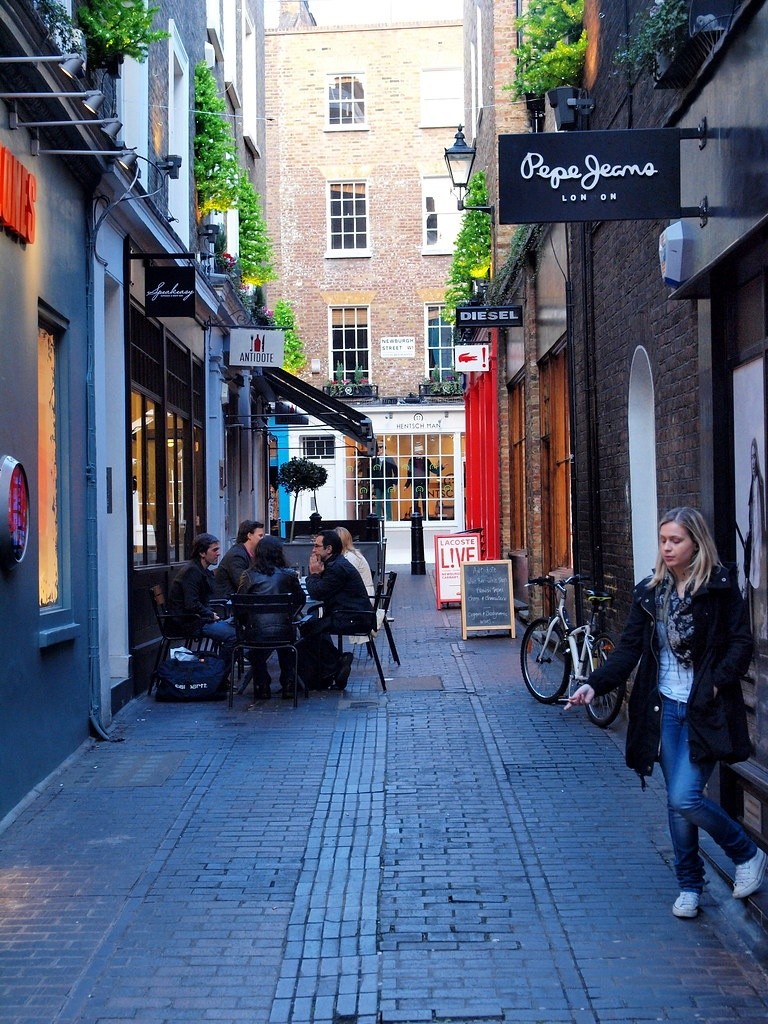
[{"x1": 313, "y1": 544, "x2": 323, "y2": 547}]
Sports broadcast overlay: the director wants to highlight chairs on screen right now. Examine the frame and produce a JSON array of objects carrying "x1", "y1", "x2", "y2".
[
  {"x1": 133, "y1": 490, "x2": 171, "y2": 546},
  {"x1": 147, "y1": 564, "x2": 401, "y2": 711}
]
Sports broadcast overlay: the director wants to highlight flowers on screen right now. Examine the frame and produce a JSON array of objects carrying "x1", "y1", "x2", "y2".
[{"x1": 218, "y1": 253, "x2": 274, "y2": 327}]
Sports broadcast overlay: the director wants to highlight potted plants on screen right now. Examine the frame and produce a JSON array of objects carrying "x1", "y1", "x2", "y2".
[{"x1": 326, "y1": 361, "x2": 464, "y2": 396}]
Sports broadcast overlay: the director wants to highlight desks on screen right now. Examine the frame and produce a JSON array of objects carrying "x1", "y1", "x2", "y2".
[{"x1": 226, "y1": 575, "x2": 322, "y2": 697}]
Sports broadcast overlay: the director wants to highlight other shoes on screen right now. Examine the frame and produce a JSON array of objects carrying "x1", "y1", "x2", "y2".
[
  {"x1": 341, "y1": 652, "x2": 353, "y2": 665},
  {"x1": 254, "y1": 683, "x2": 270, "y2": 699},
  {"x1": 234, "y1": 658, "x2": 252, "y2": 668},
  {"x1": 335, "y1": 664, "x2": 351, "y2": 690},
  {"x1": 282, "y1": 682, "x2": 295, "y2": 699}
]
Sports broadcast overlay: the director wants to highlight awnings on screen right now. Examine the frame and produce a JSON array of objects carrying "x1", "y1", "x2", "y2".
[{"x1": 224, "y1": 365, "x2": 380, "y2": 455}]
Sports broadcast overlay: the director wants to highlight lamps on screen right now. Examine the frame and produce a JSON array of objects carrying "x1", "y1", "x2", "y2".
[
  {"x1": 0, "y1": 48, "x2": 138, "y2": 170},
  {"x1": 444, "y1": 124, "x2": 495, "y2": 228},
  {"x1": 156, "y1": 155, "x2": 182, "y2": 179},
  {"x1": 198, "y1": 224, "x2": 220, "y2": 243},
  {"x1": 547, "y1": 85, "x2": 596, "y2": 131}
]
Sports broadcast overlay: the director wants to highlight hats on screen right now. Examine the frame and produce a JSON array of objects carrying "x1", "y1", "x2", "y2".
[{"x1": 415, "y1": 442, "x2": 423, "y2": 452}]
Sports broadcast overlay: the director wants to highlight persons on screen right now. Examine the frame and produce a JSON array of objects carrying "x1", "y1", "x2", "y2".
[
  {"x1": 300, "y1": 530, "x2": 376, "y2": 693},
  {"x1": 234, "y1": 534, "x2": 306, "y2": 699},
  {"x1": 165, "y1": 528, "x2": 242, "y2": 692},
  {"x1": 367, "y1": 441, "x2": 399, "y2": 521},
  {"x1": 210, "y1": 517, "x2": 265, "y2": 599},
  {"x1": 563, "y1": 503, "x2": 768, "y2": 918},
  {"x1": 334, "y1": 527, "x2": 375, "y2": 605},
  {"x1": 404, "y1": 444, "x2": 449, "y2": 520}
]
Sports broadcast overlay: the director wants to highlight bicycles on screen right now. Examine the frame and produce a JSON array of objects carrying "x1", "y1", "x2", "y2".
[{"x1": 519, "y1": 574, "x2": 628, "y2": 730}]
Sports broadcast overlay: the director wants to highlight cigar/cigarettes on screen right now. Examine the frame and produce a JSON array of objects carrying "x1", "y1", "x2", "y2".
[{"x1": 558, "y1": 698, "x2": 570, "y2": 701}]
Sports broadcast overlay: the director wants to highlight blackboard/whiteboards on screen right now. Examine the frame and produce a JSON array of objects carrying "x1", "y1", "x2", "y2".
[{"x1": 460, "y1": 559, "x2": 515, "y2": 630}]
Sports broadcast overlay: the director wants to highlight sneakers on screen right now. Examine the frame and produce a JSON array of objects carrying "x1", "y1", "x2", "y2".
[
  {"x1": 672, "y1": 891, "x2": 700, "y2": 917},
  {"x1": 733, "y1": 847, "x2": 768, "y2": 898}
]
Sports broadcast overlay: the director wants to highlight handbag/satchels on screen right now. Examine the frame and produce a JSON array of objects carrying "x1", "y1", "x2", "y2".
[
  {"x1": 298, "y1": 650, "x2": 318, "y2": 689},
  {"x1": 156, "y1": 651, "x2": 226, "y2": 700}
]
